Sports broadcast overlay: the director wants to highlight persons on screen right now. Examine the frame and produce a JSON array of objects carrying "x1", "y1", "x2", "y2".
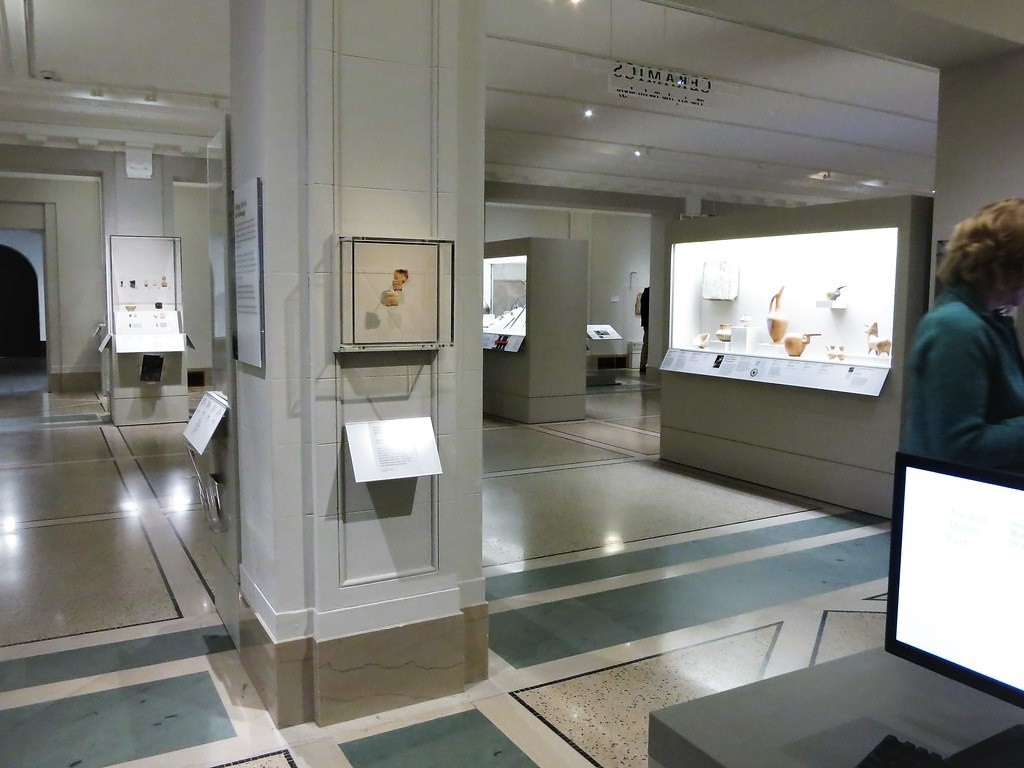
[
  {"x1": 898, "y1": 196, "x2": 1023, "y2": 486},
  {"x1": 639, "y1": 284, "x2": 651, "y2": 375}
]
